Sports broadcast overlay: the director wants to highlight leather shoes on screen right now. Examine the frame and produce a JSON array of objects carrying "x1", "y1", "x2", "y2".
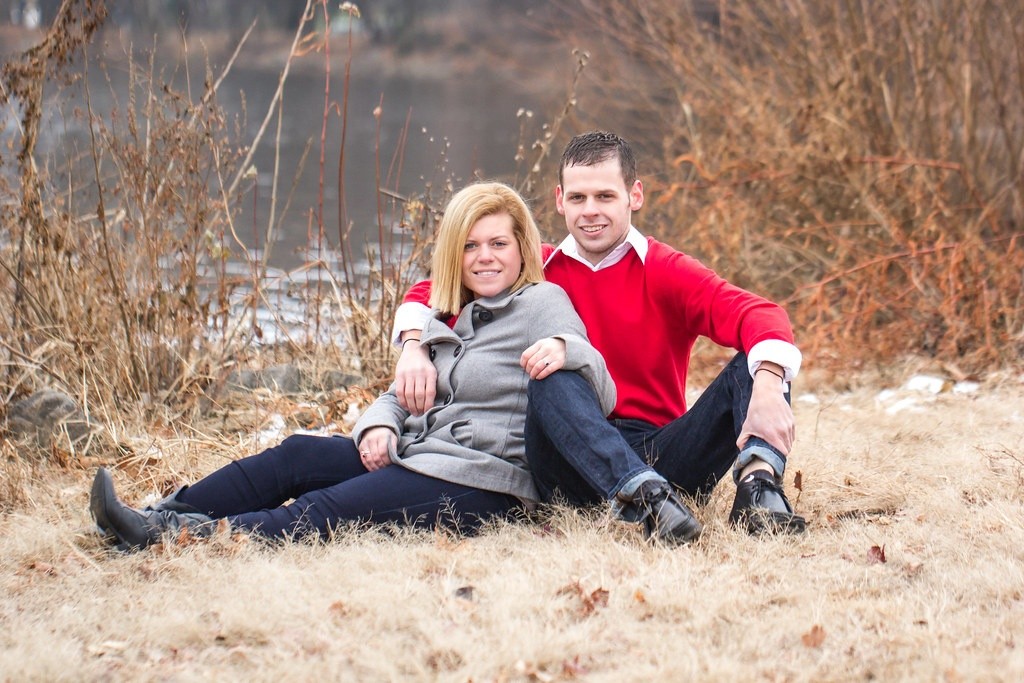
[
  {"x1": 640, "y1": 482, "x2": 701, "y2": 543},
  {"x1": 729, "y1": 479, "x2": 805, "y2": 532}
]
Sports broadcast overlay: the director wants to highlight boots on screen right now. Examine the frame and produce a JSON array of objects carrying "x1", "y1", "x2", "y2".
[
  {"x1": 97, "y1": 484, "x2": 201, "y2": 541},
  {"x1": 91, "y1": 467, "x2": 216, "y2": 556}
]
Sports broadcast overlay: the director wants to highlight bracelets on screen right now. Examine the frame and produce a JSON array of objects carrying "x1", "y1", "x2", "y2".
[
  {"x1": 754, "y1": 368, "x2": 785, "y2": 384},
  {"x1": 401, "y1": 338, "x2": 432, "y2": 352}
]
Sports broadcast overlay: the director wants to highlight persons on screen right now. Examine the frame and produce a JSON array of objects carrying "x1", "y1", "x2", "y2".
[
  {"x1": 392, "y1": 132, "x2": 808, "y2": 550},
  {"x1": 90, "y1": 182, "x2": 617, "y2": 553}
]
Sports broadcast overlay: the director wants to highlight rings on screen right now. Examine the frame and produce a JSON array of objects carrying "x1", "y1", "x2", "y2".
[
  {"x1": 361, "y1": 451, "x2": 371, "y2": 457},
  {"x1": 543, "y1": 360, "x2": 551, "y2": 366}
]
